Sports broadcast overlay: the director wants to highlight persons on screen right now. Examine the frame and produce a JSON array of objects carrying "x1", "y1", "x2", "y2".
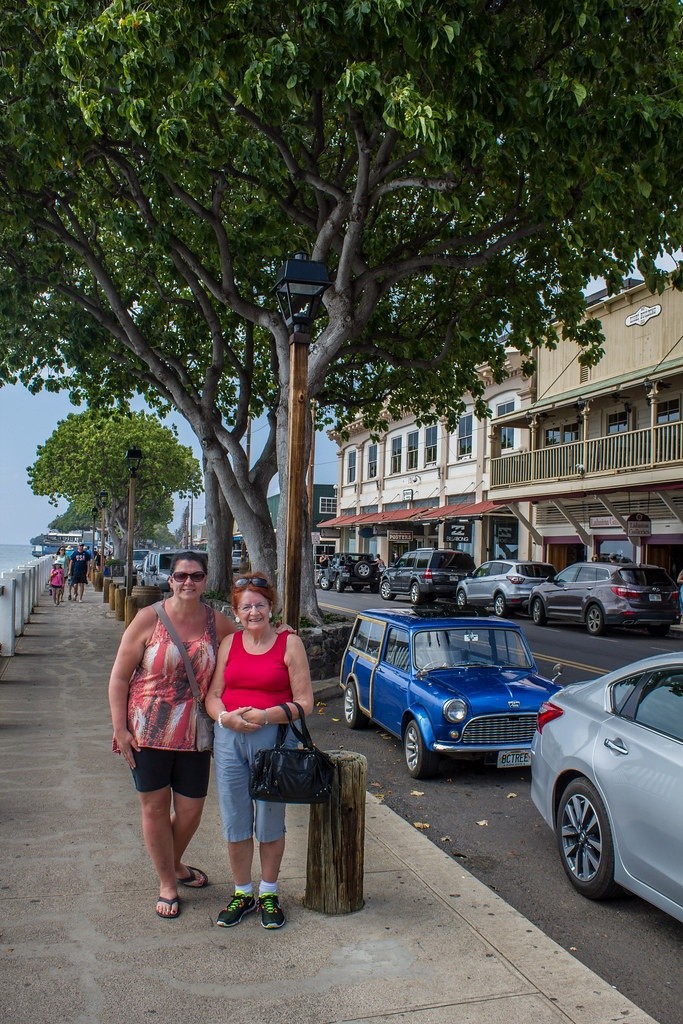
[
  {"x1": 205, "y1": 571, "x2": 314, "y2": 930},
  {"x1": 50, "y1": 543, "x2": 111, "y2": 606},
  {"x1": 375, "y1": 554, "x2": 386, "y2": 583},
  {"x1": 109, "y1": 550, "x2": 239, "y2": 918},
  {"x1": 317, "y1": 552, "x2": 330, "y2": 583},
  {"x1": 677, "y1": 570, "x2": 683, "y2": 623},
  {"x1": 591, "y1": 553, "x2": 634, "y2": 583},
  {"x1": 393, "y1": 552, "x2": 400, "y2": 564}
]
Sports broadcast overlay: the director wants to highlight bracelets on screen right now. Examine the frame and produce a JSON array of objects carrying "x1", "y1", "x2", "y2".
[
  {"x1": 218, "y1": 711, "x2": 228, "y2": 729},
  {"x1": 265, "y1": 710, "x2": 269, "y2": 726}
]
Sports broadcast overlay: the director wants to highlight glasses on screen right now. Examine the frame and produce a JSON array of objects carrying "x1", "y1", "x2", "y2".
[
  {"x1": 61, "y1": 549, "x2": 65, "y2": 551},
  {"x1": 172, "y1": 571, "x2": 206, "y2": 582},
  {"x1": 233, "y1": 577, "x2": 268, "y2": 587}
]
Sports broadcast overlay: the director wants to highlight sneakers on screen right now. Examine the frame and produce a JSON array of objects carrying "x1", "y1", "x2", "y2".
[
  {"x1": 216, "y1": 890, "x2": 256, "y2": 927},
  {"x1": 257, "y1": 893, "x2": 285, "y2": 929}
]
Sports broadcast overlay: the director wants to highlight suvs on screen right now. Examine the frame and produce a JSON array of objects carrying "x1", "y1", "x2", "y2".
[{"x1": 317, "y1": 551, "x2": 382, "y2": 593}]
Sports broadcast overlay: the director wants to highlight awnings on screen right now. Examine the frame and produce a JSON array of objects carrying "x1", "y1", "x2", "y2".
[{"x1": 316, "y1": 500, "x2": 514, "y2": 527}]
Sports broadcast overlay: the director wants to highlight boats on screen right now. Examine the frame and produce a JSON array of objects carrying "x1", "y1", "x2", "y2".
[{"x1": 30, "y1": 533, "x2": 83, "y2": 559}]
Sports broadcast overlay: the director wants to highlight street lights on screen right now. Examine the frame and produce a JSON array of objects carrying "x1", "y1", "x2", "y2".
[
  {"x1": 125, "y1": 447, "x2": 144, "y2": 592},
  {"x1": 99, "y1": 490, "x2": 109, "y2": 575},
  {"x1": 92, "y1": 506, "x2": 98, "y2": 568},
  {"x1": 275, "y1": 259, "x2": 325, "y2": 629}
]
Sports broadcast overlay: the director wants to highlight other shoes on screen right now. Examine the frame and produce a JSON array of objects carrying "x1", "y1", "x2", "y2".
[
  {"x1": 68, "y1": 595, "x2": 71, "y2": 600},
  {"x1": 79, "y1": 599, "x2": 84, "y2": 603}
]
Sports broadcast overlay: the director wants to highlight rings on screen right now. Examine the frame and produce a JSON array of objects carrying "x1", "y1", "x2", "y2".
[{"x1": 245, "y1": 724, "x2": 248, "y2": 728}]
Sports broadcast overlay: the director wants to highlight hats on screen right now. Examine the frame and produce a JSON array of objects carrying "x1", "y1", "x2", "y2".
[
  {"x1": 78, "y1": 542, "x2": 85, "y2": 546},
  {"x1": 53, "y1": 560, "x2": 64, "y2": 564}
]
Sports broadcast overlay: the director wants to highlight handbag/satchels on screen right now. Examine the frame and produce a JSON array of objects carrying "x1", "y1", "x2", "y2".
[{"x1": 248, "y1": 702, "x2": 335, "y2": 803}]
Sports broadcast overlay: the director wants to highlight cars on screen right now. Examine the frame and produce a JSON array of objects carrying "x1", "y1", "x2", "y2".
[
  {"x1": 528, "y1": 650, "x2": 683, "y2": 925},
  {"x1": 336, "y1": 606, "x2": 566, "y2": 778},
  {"x1": 136, "y1": 549, "x2": 189, "y2": 587},
  {"x1": 231, "y1": 550, "x2": 244, "y2": 570},
  {"x1": 456, "y1": 559, "x2": 558, "y2": 617},
  {"x1": 529, "y1": 561, "x2": 681, "y2": 635},
  {"x1": 379, "y1": 547, "x2": 476, "y2": 605},
  {"x1": 132, "y1": 548, "x2": 148, "y2": 574}
]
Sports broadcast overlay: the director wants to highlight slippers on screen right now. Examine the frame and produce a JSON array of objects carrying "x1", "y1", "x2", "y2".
[
  {"x1": 176, "y1": 866, "x2": 208, "y2": 888},
  {"x1": 156, "y1": 895, "x2": 181, "y2": 918}
]
